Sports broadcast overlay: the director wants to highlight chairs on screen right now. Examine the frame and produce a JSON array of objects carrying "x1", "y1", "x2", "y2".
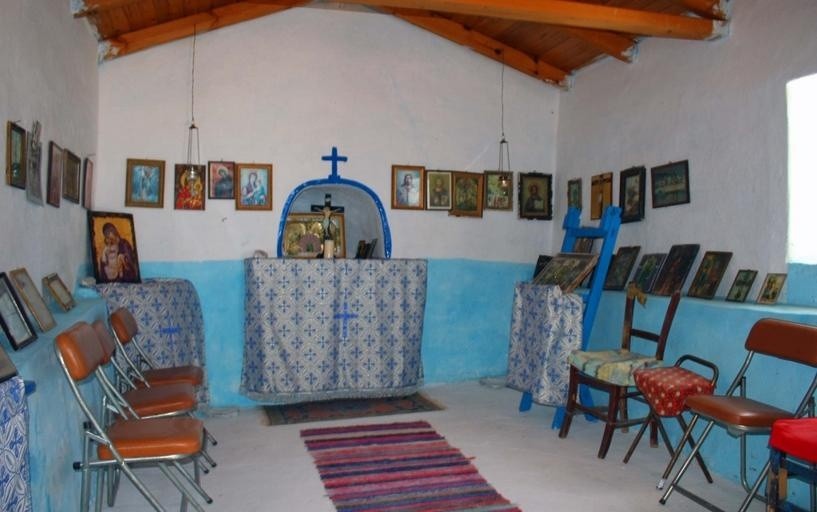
[
  {"x1": 559, "y1": 284, "x2": 678, "y2": 459},
  {"x1": 90, "y1": 319, "x2": 214, "y2": 505},
  {"x1": 622, "y1": 357, "x2": 719, "y2": 489},
  {"x1": 53, "y1": 322, "x2": 202, "y2": 511},
  {"x1": 108, "y1": 306, "x2": 217, "y2": 468},
  {"x1": 659, "y1": 319, "x2": 817, "y2": 511}
]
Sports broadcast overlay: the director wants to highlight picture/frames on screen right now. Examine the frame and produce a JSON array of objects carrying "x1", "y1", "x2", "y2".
[
  {"x1": 6, "y1": 121, "x2": 27, "y2": 189},
  {"x1": 235, "y1": 164, "x2": 273, "y2": 212},
  {"x1": 687, "y1": 251, "x2": 732, "y2": 300},
  {"x1": 0, "y1": 271, "x2": 37, "y2": 351},
  {"x1": 86, "y1": 210, "x2": 141, "y2": 284},
  {"x1": 651, "y1": 159, "x2": 690, "y2": 208},
  {"x1": 355, "y1": 239, "x2": 378, "y2": 260},
  {"x1": 632, "y1": 253, "x2": 666, "y2": 293},
  {"x1": 173, "y1": 164, "x2": 205, "y2": 211},
  {"x1": 448, "y1": 170, "x2": 482, "y2": 214},
  {"x1": 567, "y1": 178, "x2": 583, "y2": 212},
  {"x1": 62, "y1": 148, "x2": 81, "y2": 205},
  {"x1": 532, "y1": 252, "x2": 597, "y2": 294},
  {"x1": 9, "y1": 268, "x2": 57, "y2": 334},
  {"x1": 532, "y1": 255, "x2": 552, "y2": 278},
  {"x1": 482, "y1": 171, "x2": 514, "y2": 209},
  {"x1": 602, "y1": 245, "x2": 641, "y2": 290},
  {"x1": 283, "y1": 212, "x2": 346, "y2": 259},
  {"x1": 721, "y1": 270, "x2": 758, "y2": 303},
  {"x1": 425, "y1": 170, "x2": 452, "y2": 210},
  {"x1": 0, "y1": 342, "x2": 18, "y2": 382},
  {"x1": 208, "y1": 160, "x2": 230, "y2": 198},
  {"x1": 26, "y1": 120, "x2": 43, "y2": 205},
  {"x1": 46, "y1": 140, "x2": 64, "y2": 208},
  {"x1": 391, "y1": 166, "x2": 425, "y2": 210},
  {"x1": 650, "y1": 244, "x2": 700, "y2": 297},
  {"x1": 124, "y1": 158, "x2": 164, "y2": 208},
  {"x1": 618, "y1": 166, "x2": 646, "y2": 223},
  {"x1": 590, "y1": 171, "x2": 613, "y2": 220},
  {"x1": 518, "y1": 172, "x2": 553, "y2": 219},
  {"x1": 82, "y1": 158, "x2": 93, "y2": 209},
  {"x1": 756, "y1": 273, "x2": 787, "y2": 306},
  {"x1": 43, "y1": 274, "x2": 77, "y2": 313}
]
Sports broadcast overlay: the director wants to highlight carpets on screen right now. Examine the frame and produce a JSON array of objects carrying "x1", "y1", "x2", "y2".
[
  {"x1": 262, "y1": 391, "x2": 446, "y2": 424},
  {"x1": 299, "y1": 419, "x2": 523, "y2": 512}
]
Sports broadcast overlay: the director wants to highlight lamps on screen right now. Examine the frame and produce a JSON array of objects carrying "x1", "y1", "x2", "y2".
[
  {"x1": 184, "y1": 20, "x2": 201, "y2": 196},
  {"x1": 498, "y1": 47, "x2": 510, "y2": 195}
]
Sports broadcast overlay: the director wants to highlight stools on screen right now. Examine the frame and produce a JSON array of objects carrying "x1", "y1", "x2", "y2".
[{"x1": 767, "y1": 416, "x2": 816, "y2": 511}]
